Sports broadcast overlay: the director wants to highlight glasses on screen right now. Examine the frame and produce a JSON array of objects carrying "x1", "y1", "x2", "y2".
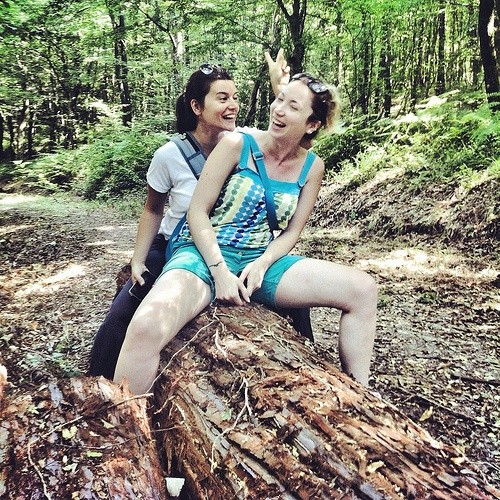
[
  {"x1": 199, "y1": 64, "x2": 226, "y2": 76},
  {"x1": 292, "y1": 73, "x2": 329, "y2": 95}
]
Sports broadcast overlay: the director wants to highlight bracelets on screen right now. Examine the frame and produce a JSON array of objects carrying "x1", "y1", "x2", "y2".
[{"x1": 208, "y1": 260, "x2": 223, "y2": 268}]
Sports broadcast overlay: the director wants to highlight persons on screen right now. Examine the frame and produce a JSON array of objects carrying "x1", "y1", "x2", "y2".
[
  {"x1": 87, "y1": 47, "x2": 315, "y2": 381},
  {"x1": 111, "y1": 72, "x2": 378, "y2": 395}
]
